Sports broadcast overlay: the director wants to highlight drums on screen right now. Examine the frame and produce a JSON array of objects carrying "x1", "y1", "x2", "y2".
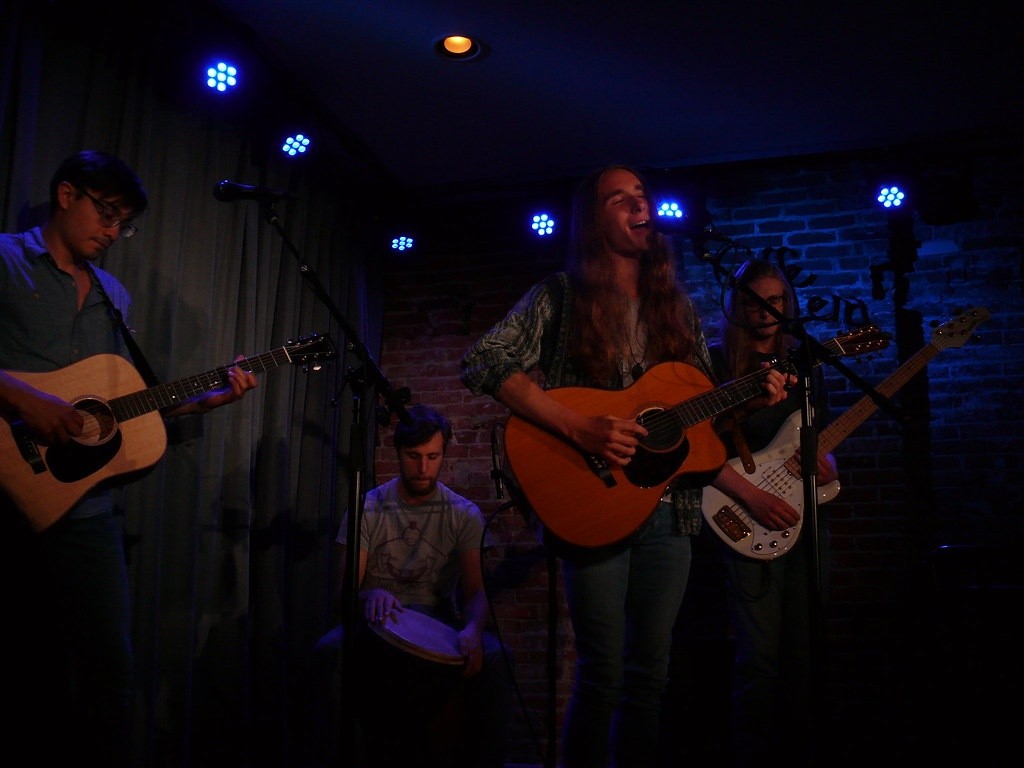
[{"x1": 366, "y1": 606, "x2": 461, "y2": 665}]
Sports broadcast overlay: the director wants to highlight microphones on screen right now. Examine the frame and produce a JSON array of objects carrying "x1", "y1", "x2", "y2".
[
  {"x1": 491, "y1": 431, "x2": 503, "y2": 499},
  {"x1": 654, "y1": 217, "x2": 732, "y2": 244},
  {"x1": 212, "y1": 179, "x2": 293, "y2": 202}
]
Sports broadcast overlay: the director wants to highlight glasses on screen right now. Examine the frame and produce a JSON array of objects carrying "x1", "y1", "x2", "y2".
[{"x1": 77, "y1": 187, "x2": 136, "y2": 240}]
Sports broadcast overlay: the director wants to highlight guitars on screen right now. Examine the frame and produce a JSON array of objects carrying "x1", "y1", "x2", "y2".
[
  {"x1": 699, "y1": 308, "x2": 990, "y2": 559},
  {"x1": 487, "y1": 328, "x2": 890, "y2": 563},
  {"x1": 0, "y1": 331, "x2": 330, "y2": 548}
]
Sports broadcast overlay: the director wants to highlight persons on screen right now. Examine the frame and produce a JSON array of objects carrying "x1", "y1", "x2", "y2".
[
  {"x1": 332, "y1": 407, "x2": 513, "y2": 768},
  {"x1": 460, "y1": 163, "x2": 799, "y2": 768},
  {"x1": 708, "y1": 260, "x2": 840, "y2": 767},
  {"x1": 0, "y1": 150, "x2": 259, "y2": 768}
]
